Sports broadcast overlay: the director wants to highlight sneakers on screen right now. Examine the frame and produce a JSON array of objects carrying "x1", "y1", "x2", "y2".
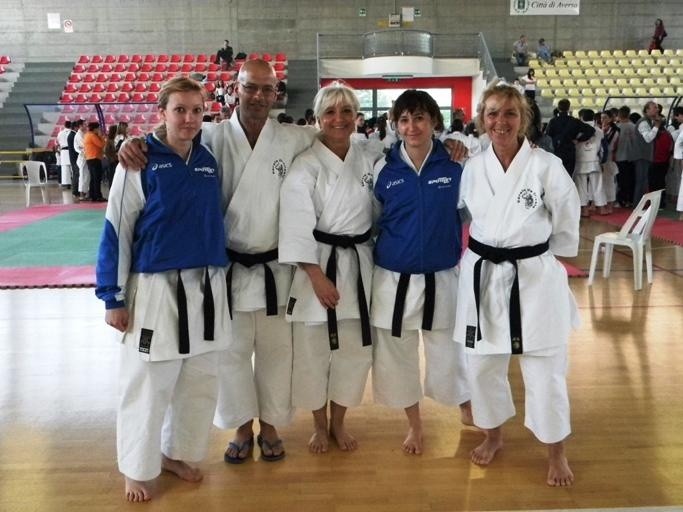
[
  {"x1": 71, "y1": 195, "x2": 107, "y2": 202},
  {"x1": 579, "y1": 199, "x2": 665, "y2": 217}
]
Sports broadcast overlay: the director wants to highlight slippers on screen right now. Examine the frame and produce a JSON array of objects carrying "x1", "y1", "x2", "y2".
[
  {"x1": 222, "y1": 431, "x2": 254, "y2": 464},
  {"x1": 255, "y1": 429, "x2": 285, "y2": 461}
]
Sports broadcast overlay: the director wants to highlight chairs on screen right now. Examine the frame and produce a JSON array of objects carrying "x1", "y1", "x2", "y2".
[
  {"x1": 588, "y1": 187, "x2": 666, "y2": 290},
  {"x1": 527, "y1": 49, "x2": 683, "y2": 117},
  {"x1": 45, "y1": 52, "x2": 286, "y2": 156},
  {"x1": 19, "y1": 161, "x2": 63, "y2": 209},
  {"x1": 0, "y1": 55, "x2": 11, "y2": 64},
  {"x1": 0, "y1": 66, "x2": 6, "y2": 74}
]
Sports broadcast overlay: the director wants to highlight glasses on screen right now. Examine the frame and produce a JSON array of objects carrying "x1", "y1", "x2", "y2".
[{"x1": 237, "y1": 81, "x2": 276, "y2": 96}]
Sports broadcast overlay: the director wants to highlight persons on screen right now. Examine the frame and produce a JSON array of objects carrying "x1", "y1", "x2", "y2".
[
  {"x1": 652, "y1": 18, "x2": 664, "y2": 53},
  {"x1": 115, "y1": 59, "x2": 369, "y2": 464},
  {"x1": 214, "y1": 39, "x2": 233, "y2": 67},
  {"x1": 93, "y1": 74, "x2": 230, "y2": 503},
  {"x1": 276, "y1": 81, "x2": 471, "y2": 453},
  {"x1": 372, "y1": 88, "x2": 540, "y2": 456},
  {"x1": 53, "y1": 76, "x2": 683, "y2": 219},
  {"x1": 512, "y1": 33, "x2": 529, "y2": 65},
  {"x1": 452, "y1": 82, "x2": 580, "y2": 489},
  {"x1": 520, "y1": 68, "x2": 537, "y2": 98},
  {"x1": 534, "y1": 37, "x2": 554, "y2": 65}
]
